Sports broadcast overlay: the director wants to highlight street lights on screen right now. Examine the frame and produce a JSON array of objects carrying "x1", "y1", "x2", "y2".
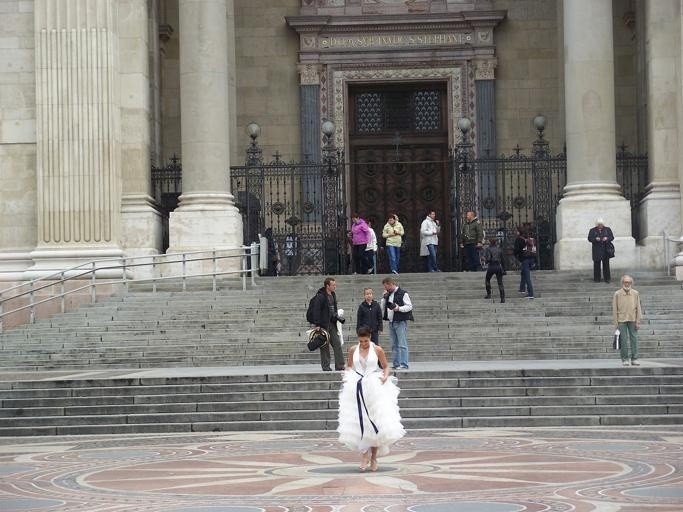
[
  {"x1": 245, "y1": 120, "x2": 265, "y2": 278},
  {"x1": 322, "y1": 119, "x2": 345, "y2": 275},
  {"x1": 458, "y1": 117, "x2": 476, "y2": 237},
  {"x1": 532, "y1": 114, "x2": 554, "y2": 270}
]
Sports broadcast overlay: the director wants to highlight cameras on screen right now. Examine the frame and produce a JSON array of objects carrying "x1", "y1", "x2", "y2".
[
  {"x1": 331, "y1": 312, "x2": 346, "y2": 324},
  {"x1": 386, "y1": 301, "x2": 396, "y2": 309}
]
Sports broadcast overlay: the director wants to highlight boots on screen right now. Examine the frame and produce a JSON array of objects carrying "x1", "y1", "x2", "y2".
[
  {"x1": 499, "y1": 289, "x2": 505, "y2": 303},
  {"x1": 485, "y1": 285, "x2": 491, "y2": 299}
]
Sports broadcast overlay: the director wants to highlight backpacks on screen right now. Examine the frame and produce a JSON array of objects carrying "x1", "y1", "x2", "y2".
[
  {"x1": 520, "y1": 236, "x2": 537, "y2": 254},
  {"x1": 306, "y1": 292, "x2": 318, "y2": 322}
]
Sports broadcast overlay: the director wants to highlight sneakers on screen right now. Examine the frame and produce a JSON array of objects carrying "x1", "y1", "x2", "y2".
[
  {"x1": 323, "y1": 367, "x2": 332, "y2": 371},
  {"x1": 368, "y1": 267, "x2": 373, "y2": 273},
  {"x1": 632, "y1": 360, "x2": 640, "y2": 365},
  {"x1": 518, "y1": 290, "x2": 525, "y2": 293},
  {"x1": 623, "y1": 361, "x2": 630, "y2": 366},
  {"x1": 524, "y1": 295, "x2": 534, "y2": 299}
]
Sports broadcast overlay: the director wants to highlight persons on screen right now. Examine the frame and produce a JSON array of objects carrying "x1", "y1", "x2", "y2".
[
  {"x1": 612, "y1": 274, "x2": 641, "y2": 365},
  {"x1": 258, "y1": 227, "x2": 281, "y2": 276},
  {"x1": 340, "y1": 207, "x2": 554, "y2": 303},
  {"x1": 380, "y1": 276, "x2": 415, "y2": 370},
  {"x1": 335, "y1": 324, "x2": 407, "y2": 471},
  {"x1": 307, "y1": 277, "x2": 346, "y2": 371},
  {"x1": 356, "y1": 287, "x2": 383, "y2": 369},
  {"x1": 588, "y1": 216, "x2": 615, "y2": 283}
]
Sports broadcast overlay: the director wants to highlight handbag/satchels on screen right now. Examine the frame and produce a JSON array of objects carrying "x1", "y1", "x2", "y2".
[
  {"x1": 308, "y1": 332, "x2": 326, "y2": 351},
  {"x1": 606, "y1": 242, "x2": 615, "y2": 258},
  {"x1": 613, "y1": 329, "x2": 621, "y2": 350}
]
